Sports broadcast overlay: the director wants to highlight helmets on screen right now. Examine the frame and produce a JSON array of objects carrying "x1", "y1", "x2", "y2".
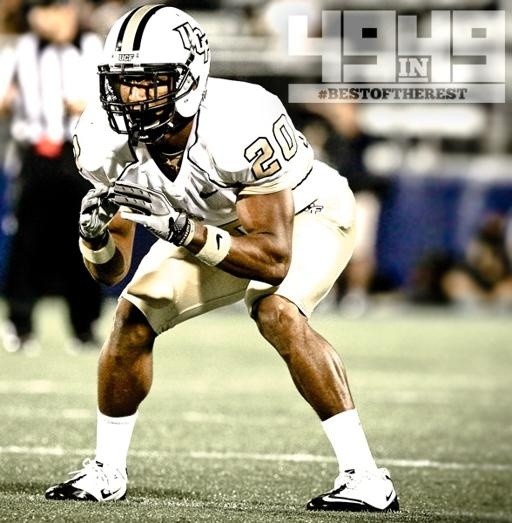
[{"x1": 94, "y1": 3, "x2": 214, "y2": 152}]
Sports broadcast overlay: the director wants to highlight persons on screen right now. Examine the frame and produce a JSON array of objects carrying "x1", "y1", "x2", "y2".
[
  {"x1": 2, "y1": 0, "x2": 512, "y2": 348},
  {"x1": 44, "y1": 2, "x2": 400, "y2": 514}
]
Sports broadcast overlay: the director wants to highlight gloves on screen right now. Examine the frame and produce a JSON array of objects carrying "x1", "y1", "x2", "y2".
[{"x1": 76, "y1": 179, "x2": 196, "y2": 254}]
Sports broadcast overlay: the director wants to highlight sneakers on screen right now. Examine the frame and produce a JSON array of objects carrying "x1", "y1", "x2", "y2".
[
  {"x1": 44, "y1": 457, "x2": 129, "y2": 505},
  {"x1": 303, "y1": 467, "x2": 401, "y2": 514}
]
